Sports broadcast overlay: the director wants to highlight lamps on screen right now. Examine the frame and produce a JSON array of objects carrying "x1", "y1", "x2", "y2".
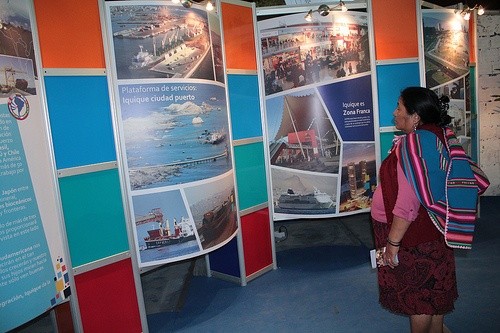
[
  {"x1": 460, "y1": 4, "x2": 485, "y2": 20},
  {"x1": 172, "y1": 0, "x2": 213, "y2": 11},
  {"x1": 305, "y1": 0, "x2": 348, "y2": 21}
]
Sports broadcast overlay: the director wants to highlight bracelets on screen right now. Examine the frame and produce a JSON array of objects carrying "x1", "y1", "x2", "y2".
[{"x1": 385, "y1": 236, "x2": 400, "y2": 246}]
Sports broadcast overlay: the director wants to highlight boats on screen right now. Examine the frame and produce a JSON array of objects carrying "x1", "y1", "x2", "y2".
[
  {"x1": 144, "y1": 216, "x2": 196, "y2": 250},
  {"x1": 210, "y1": 127, "x2": 227, "y2": 144},
  {"x1": 276, "y1": 186, "x2": 334, "y2": 210}
]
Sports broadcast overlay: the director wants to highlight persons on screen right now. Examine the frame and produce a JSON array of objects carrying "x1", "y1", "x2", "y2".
[{"x1": 371, "y1": 87, "x2": 460, "y2": 333}]
